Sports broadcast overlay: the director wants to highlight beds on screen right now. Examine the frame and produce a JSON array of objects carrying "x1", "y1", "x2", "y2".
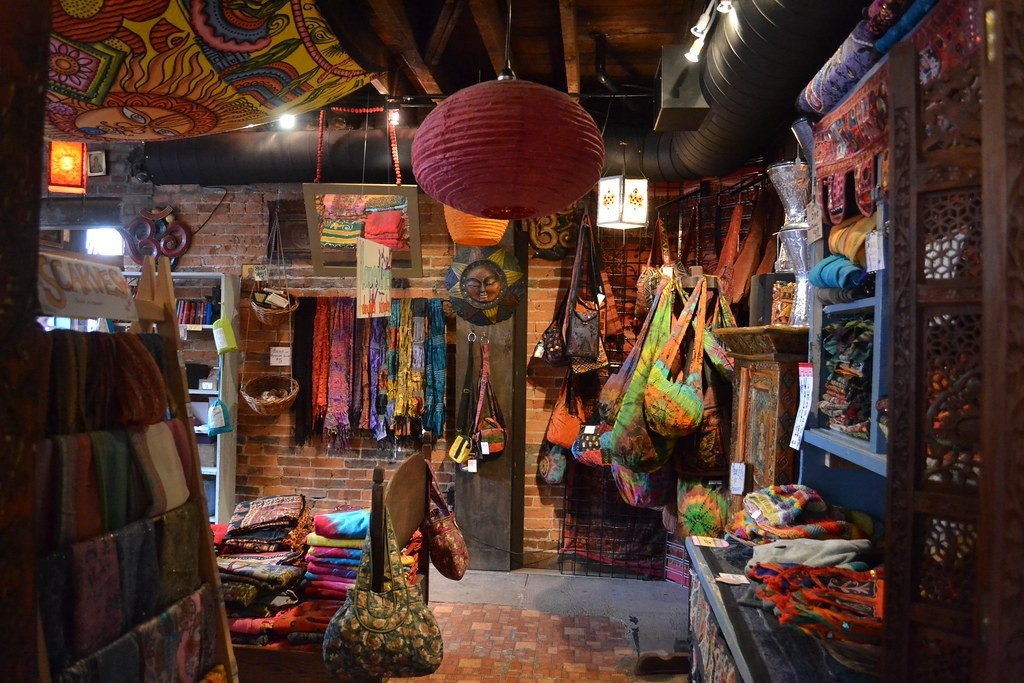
[{"x1": 231, "y1": 430, "x2": 434, "y2": 683}]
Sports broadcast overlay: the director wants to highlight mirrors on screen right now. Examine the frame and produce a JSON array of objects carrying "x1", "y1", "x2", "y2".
[{"x1": 302, "y1": 178, "x2": 424, "y2": 278}]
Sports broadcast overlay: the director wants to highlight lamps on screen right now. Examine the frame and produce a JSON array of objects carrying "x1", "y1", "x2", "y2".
[
  {"x1": 596, "y1": 139, "x2": 651, "y2": 238},
  {"x1": 47, "y1": 141, "x2": 88, "y2": 210}
]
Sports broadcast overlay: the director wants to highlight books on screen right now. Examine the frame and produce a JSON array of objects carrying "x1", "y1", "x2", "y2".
[{"x1": 176, "y1": 297, "x2": 214, "y2": 325}]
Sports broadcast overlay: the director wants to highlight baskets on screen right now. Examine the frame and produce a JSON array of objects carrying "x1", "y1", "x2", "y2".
[
  {"x1": 821, "y1": 634, "x2": 882, "y2": 677},
  {"x1": 242, "y1": 375, "x2": 301, "y2": 414},
  {"x1": 251, "y1": 291, "x2": 300, "y2": 326}
]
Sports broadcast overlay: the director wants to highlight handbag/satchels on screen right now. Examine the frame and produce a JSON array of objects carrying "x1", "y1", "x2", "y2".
[
  {"x1": 449, "y1": 417, "x2": 509, "y2": 472},
  {"x1": 323, "y1": 503, "x2": 444, "y2": 680},
  {"x1": 425, "y1": 459, "x2": 469, "y2": 583},
  {"x1": 534, "y1": 266, "x2": 738, "y2": 546}
]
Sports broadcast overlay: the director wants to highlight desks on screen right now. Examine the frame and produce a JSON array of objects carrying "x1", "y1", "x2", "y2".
[{"x1": 684, "y1": 532, "x2": 856, "y2": 683}]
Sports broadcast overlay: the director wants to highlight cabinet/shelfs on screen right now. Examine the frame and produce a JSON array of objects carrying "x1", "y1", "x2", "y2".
[
  {"x1": 792, "y1": 151, "x2": 983, "y2": 533},
  {"x1": 120, "y1": 271, "x2": 241, "y2": 527}
]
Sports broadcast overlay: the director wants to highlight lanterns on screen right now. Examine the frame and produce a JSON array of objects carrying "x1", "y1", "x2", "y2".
[
  {"x1": 412, "y1": 82, "x2": 604, "y2": 221},
  {"x1": 597, "y1": 176, "x2": 649, "y2": 231},
  {"x1": 49, "y1": 141, "x2": 89, "y2": 194},
  {"x1": 444, "y1": 205, "x2": 509, "y2": 245}
]
